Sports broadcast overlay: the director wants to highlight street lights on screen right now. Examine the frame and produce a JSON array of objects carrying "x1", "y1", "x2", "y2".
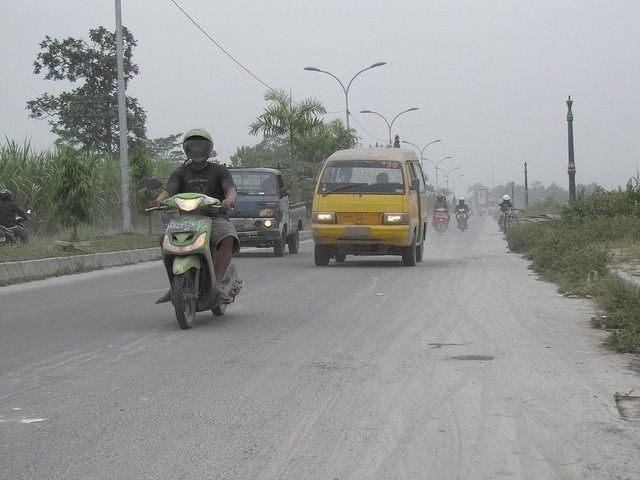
[
  {"x1": 360, "y1": 108, "x2": 419, "y2": 147},
  {"x1": 401, "y1": 139, "x2": 442, "y2": 168},
  {"x1": 421, "y1": 157, "x2": 452, "y2": 191},
  {"x1": 304, "y1": 61, "x2": 387, "y2": 131},
  {"x1": 442, "y1": 174, "x2": 464, "y2": 201},
  {"x1": 436, "y1": 167, "x2": 459, "y2": 191}
]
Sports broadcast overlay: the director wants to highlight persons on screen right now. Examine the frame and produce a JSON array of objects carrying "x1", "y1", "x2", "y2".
[
  {"x1": 146, "y1": 127, "x2": 241, "y2": 304},
  {"x1": 375, "y1": 173, "x2": 390, "y2": 186},
  {"x1": 478, "y1": 207, "x2": 483, "y2": 215},
  {"x1": 432, "y1": 195, "x2": 449, "y2": 229},
  {"x1": 498, "y1": 195, "x2": 515, "y2": 232},
  {"x1": 454, "y1": 197, "x2": 470, "y2": 226},
  {"x1": 1, "y1": 188, "x2": 30, "y2": 246}
]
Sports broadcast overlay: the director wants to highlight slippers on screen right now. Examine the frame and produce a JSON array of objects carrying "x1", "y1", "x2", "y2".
[
  {"x1": 155, "y1": 295, "x2": 170, "y2": 304},
  {"x1": 216, "y1": 292, "x2": 232, "y2": 301}
]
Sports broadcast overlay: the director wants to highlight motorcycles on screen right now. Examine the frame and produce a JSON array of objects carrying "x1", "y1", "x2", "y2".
[
  {"x1": 497, "y1": 208, "x2": 517, "y2": 234},
  {"x1": 455, "y1": 209, "x2": 469, "y2": 230},
  {"x1": 146, "y1": 176, "x2": 245, "y2": 330},
  {"x1": 432, "y1": 207, "x2": 450, "y2": 235},
  {"x1": 1, "y1": 209, "x2": 32, "y2": 245}
]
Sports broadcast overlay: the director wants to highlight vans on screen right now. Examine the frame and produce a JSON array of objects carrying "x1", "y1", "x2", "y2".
[{"x1": 312, "y1": 148, "x2": 426, "y2": 266}]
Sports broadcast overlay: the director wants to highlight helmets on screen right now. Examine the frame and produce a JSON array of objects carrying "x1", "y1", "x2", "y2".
[
  {"x1": 459, "y1": 197, "x2": 465, "y2": 204},
  {"x1": 437, "y1": 194, "x2": 444, "y2": 202},
  {"x1": 0, "y1": 188, "x2": 13, "y2": 201},
  {"x1": 183, "y1": 128, "x2": 213, "y2": 164},
  {"x1": 503, "y1": 194, "x2": 510, "y2": 201}
]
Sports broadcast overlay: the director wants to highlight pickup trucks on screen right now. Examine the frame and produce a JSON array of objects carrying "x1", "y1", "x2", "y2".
[{"x1": 220, "y1": 163, "x2": 307, "y2": 257}]
